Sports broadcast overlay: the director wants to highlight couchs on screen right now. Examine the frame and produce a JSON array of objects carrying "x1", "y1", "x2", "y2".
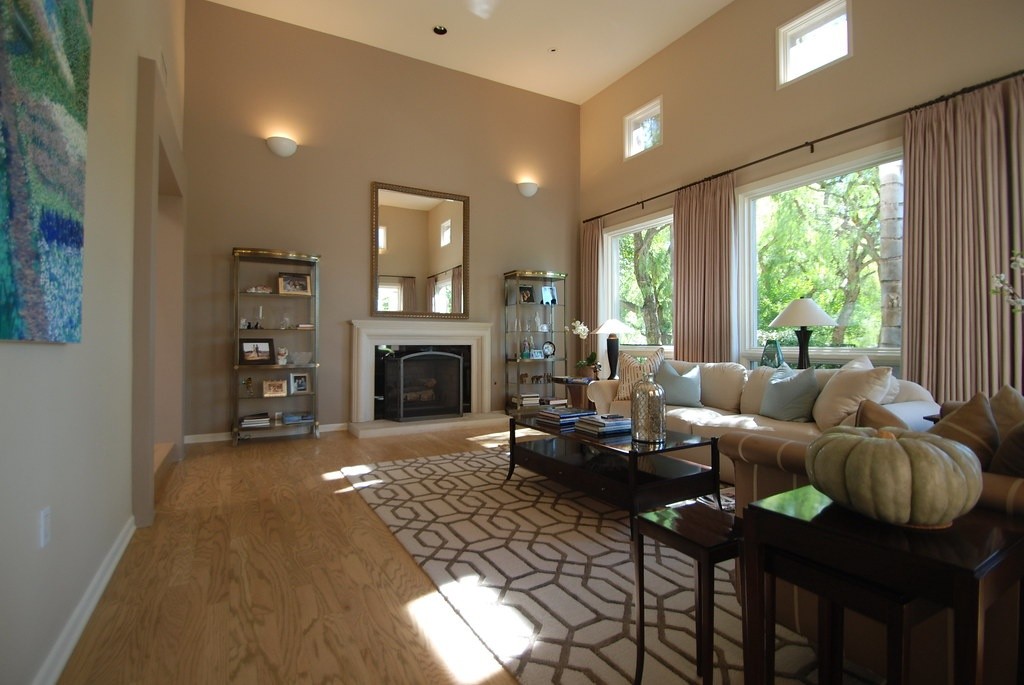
[
  {"x1": 587, "y1": 361, "x2": 943, "y2": 487},
  {"x1": 750, "y1": 396, "x2": 1024, "y2": 685}
]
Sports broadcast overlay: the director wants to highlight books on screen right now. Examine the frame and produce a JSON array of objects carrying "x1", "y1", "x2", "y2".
[
  {"x1": 511, "y1": 393, "x2": 568, "y2": 406},
  {"x1": 282, "y1": 412, "x2": 314, "y2": 423},
  {"x1": 536, "y1": 408, "x2": 631, "y2": 437},
  {"x1": 240, "y1": 412, "x2": 271, "y2": 427}
]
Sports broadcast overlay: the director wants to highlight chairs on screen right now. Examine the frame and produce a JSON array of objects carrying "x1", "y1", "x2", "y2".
[{"x1": 629, "y1": 436, "x2": 749, "y2": 685}]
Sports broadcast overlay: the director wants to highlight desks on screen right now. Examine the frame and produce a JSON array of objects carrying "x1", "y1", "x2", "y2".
[{"x1": 743, "y1": 483, "x2": 1024, "y2": 685}]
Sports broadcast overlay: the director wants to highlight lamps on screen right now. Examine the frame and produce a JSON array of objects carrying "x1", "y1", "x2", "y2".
[
  {"x1": 516, "y1": 183, "x2": 538, "y2": 196},
  {"x1": 768, "y1": 297, "x2": 839, "y2": 369},
  {"x1": 266, "y1": 136, "x2": 298, "y2": 158},
  {"x1": 590, "y1": 319, "x2": 635, "y2": 380}
]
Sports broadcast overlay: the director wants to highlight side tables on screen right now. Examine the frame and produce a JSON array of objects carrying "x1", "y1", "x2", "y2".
[{"x1": 570, "y1": 379, "x2": 598, "y2": 411}]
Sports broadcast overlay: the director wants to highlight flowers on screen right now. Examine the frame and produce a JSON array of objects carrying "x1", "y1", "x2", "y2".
[
  {"x1": 989, "y1": 250, "x2": 1024, "y2": 315},
  {"x1": 572, "y1": 320, "x2": 602, "y2": 368}
]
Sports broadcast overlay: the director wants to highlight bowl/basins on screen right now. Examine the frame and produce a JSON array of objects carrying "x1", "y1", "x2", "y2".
[{"x1": 290, "y1": 352, "x2": 312, "y2": 365}]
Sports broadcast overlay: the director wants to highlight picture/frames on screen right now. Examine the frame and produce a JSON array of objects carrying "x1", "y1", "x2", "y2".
[
  {"x1": 290, "y1": 372, "x2": 311, "y2": 396},
  {"x1": 278, "y1": 272, "x2": 311, "y2": 294},
  {"x1": 262, "y1": 379, "x2": 287, "y2": 397},
  {"x1": 239, "y1": 338, "x2": 276, "y2": 365},
  {"x1": 519, "y1": 284, "x2": 537, "y2": 305}
]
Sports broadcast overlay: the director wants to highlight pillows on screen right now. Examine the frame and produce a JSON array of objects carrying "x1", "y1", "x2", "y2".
[
  {"x1": 613, "y1": 348, "x2": 665, "y2": 401},
  {"x1": 854, "y1": 384, "x2": 1024, "y2": 477},
  {"x1": 812, "y1": 355, "x2": 899, "y2": 434},
  {"x1": 757, "y1": 362, "x2": 820, "y2": 422},
  {"x1": 654, "y1": 360, "x2": 704, "y2": 408}
]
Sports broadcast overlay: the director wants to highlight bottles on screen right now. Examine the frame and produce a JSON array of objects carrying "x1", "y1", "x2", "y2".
[
  {"x1": 631, "y1": 373, "x2": 667, "y2": 443},
  {"x1": 761, "y1": 340, "x2": 784, "y2": 368}
]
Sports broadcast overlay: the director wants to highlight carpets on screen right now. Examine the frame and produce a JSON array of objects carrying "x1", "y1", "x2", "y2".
[{"x1": 341, "y1": 445, "x2": 864, "y2": 685}]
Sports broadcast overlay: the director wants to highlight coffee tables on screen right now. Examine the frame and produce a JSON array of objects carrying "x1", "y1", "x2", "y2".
[{"x1": 507, "y1": 408, "x2": 724, "y2": 542}]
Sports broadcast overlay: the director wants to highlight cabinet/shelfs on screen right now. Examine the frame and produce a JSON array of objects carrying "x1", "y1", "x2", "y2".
[
  {"x1": 232, "y1": 247, "x2": 320, "y2": 442},
  {"x1": 502, "y1": 270, "x2": 568, "y2": 414}
]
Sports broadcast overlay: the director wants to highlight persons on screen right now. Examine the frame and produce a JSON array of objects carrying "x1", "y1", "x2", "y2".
[
  {"x1": 271, "y1": 384, "x2": 275, "y2": 392},
  {"x1": 295, "y1": 378, "x2": 306, "y2": 391},
  {"x1": 250, "y1": 345, "x2": 259, "y2": 357},
  {"x1": 285, "y1": 279, "x2": 303, "y2": 293}
]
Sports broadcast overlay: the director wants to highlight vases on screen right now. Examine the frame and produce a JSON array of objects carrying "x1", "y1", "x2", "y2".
[{"x1": 591, "y1": 365, "x2": 600, "y2": 380}]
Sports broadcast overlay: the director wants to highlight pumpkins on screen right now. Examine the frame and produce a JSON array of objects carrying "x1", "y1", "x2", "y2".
[{"x1": 804, "y1": 425, "x2": 984, "y2": 526}]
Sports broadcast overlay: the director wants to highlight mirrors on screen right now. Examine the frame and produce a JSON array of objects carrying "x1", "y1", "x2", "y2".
[{"x1": 368, "y1": 182, "x2": 471, "y2": 320}]
